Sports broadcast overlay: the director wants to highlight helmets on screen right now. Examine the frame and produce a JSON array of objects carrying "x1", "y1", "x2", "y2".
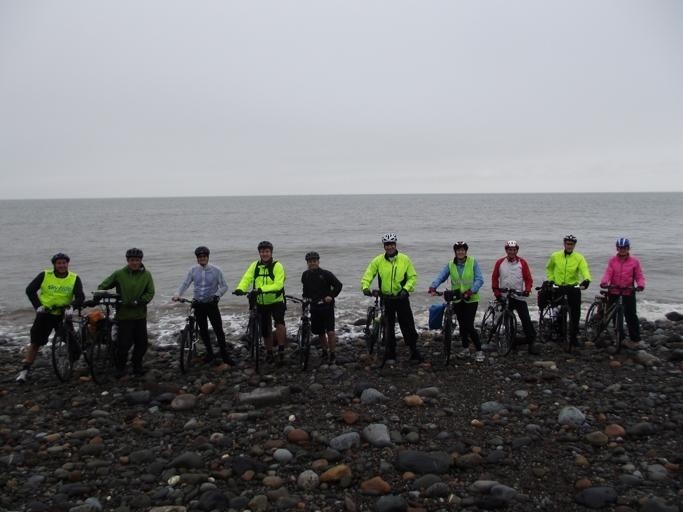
[
  {"x1": 51, "y1": 253, "x2": 71, "y2": 264},
  {"x1": 381, "y1": 233, "x2": 397, "y2": 244},
  {"x1": 305, "y1": 251, "x2": 321, "y2": 261},
  {"x1": 125, "y1": 247, "x2": 143, "y2": 259},
  {"x1": 257, "y1": 241, "x2": 273, "y2": 251},
  {"x1": 505, "y1": 240, "x2": 520, "y2": 251},
  {"x1": 453, "y1": 241, "x2": 468, "y2": 251},
  {"x1": 563, "y1": 234, "x2": 577, "y2": 243},
  {"x1": 194, "y1": 246, "x2": 210, "y2": 256},
  {"x1": 615, "y1": 238, "x2": 629, "y2": 249}
]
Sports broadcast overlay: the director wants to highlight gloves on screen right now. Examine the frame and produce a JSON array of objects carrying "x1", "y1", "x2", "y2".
[
  {"x1": 363, "y1": 288, "x2": 372, "y2": 296},
  {"x1": 462, "y1": 288, "x2": 472, "y2": 300},
  {"x1": 427, "y1": 288, "x2": 437, "y2": 296},
  {"x1": 636, "y1": 285, "x2": 644, "y2": 292},
  {"x1": 400, "y1": 290, "x2": 408, "y2": 297},
  {"x1": 235, "y1": 289, "x2": 243, "y2": 296},
  {"x1": 600, "y1": 282, "x2": 608, "y2": 288},
  {"x1": 36, "y1": 306, "x2": 45, "y2": 313},
  {"x1": 64, "y1": 305, "x2": 73, "y2": 317},
  {"x1": 579, "y1": 279, "x2": 590, "y2": 289}
]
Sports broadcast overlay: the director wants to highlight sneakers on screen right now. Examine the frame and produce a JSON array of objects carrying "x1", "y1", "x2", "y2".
[
  {"x1": 475, "y1": 351, "x2": 485, "y2": 362},
  {"x1": 322, "y1": 354, "x2": 329, "y2": 363},
  {"x1": 455, "y1": 348, "x2": 470, "y2": 357},
  {"x1": 329, "y1": 356, "x2": 336, "y2": 364},
  {"x1": 263, "y1": 358, "x2": 275, "y2": 372},
  {"x1": 223, "y1": 356, "x2": 236, "y2": 367},
  {"x1": 15, "y1": 368, "x2": 28, "y2": 383},
  {"x1": 201, "y1": 353, "x2": 213, "y2": 364}
]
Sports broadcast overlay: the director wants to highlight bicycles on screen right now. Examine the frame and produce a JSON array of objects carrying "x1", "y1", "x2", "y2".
[
  {"x1": 585, "y1": 283, "x2": 644, "y2": 350},
  {"x1": 231, "y1": 290, "x2": 272, "y2": 372},
  {"x1": 45, "y1": 300, "x2": 95, "y2": 384},
  {"x1": 364, "y1": 289, "x2": 407, "y2": 369},
  {"x1": 480, "y1": 288, "x2": 528, "y2": 356},
  {"x1": 86, "y1": 299, "x2": 138, "y2": 385},
  {"x1": 431, "y1": 288, "x2": 471, "y2": 365},
  {"x1": 172, "y1": 295, "x2": 218, "y2": 374},
  {"x1": 538, "y1": 282, "x2": 579, "y2": 349},
  {"x1": 284, "y1": 294, "x2": 333, "y2": 371}
]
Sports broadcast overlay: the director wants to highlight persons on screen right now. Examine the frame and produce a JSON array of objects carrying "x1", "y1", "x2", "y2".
[
  {"x1": 234, "y1": 241, "x2": 288, "y2": 365},
  {"x1": 96, "y1": 248, "x2": 155, "y2": 376},
  {"x1": 600, "y1": 239, "x2": 647, "y2": 349},
  {"x1": 547, "y1": 236, "x2": 591, "y2": 350},
  {"x1": 361, "y1": 233, "x2": 423, "y2": 363},
  {"x1": 171, "y1": 247, "x2": 229, "y2": 365},
  {"x1": 429, "y1": 243, "x2": 485, "y2": 359},
  {"x1": 16, "y1": 254, "x2": 84, "y2": 382},
  {"x1": 493, "y1": 241, "x2": 537, "y2": 356},
  {"x1": 301, "y1": 252, "x2": 342, "y2": 364}
]
